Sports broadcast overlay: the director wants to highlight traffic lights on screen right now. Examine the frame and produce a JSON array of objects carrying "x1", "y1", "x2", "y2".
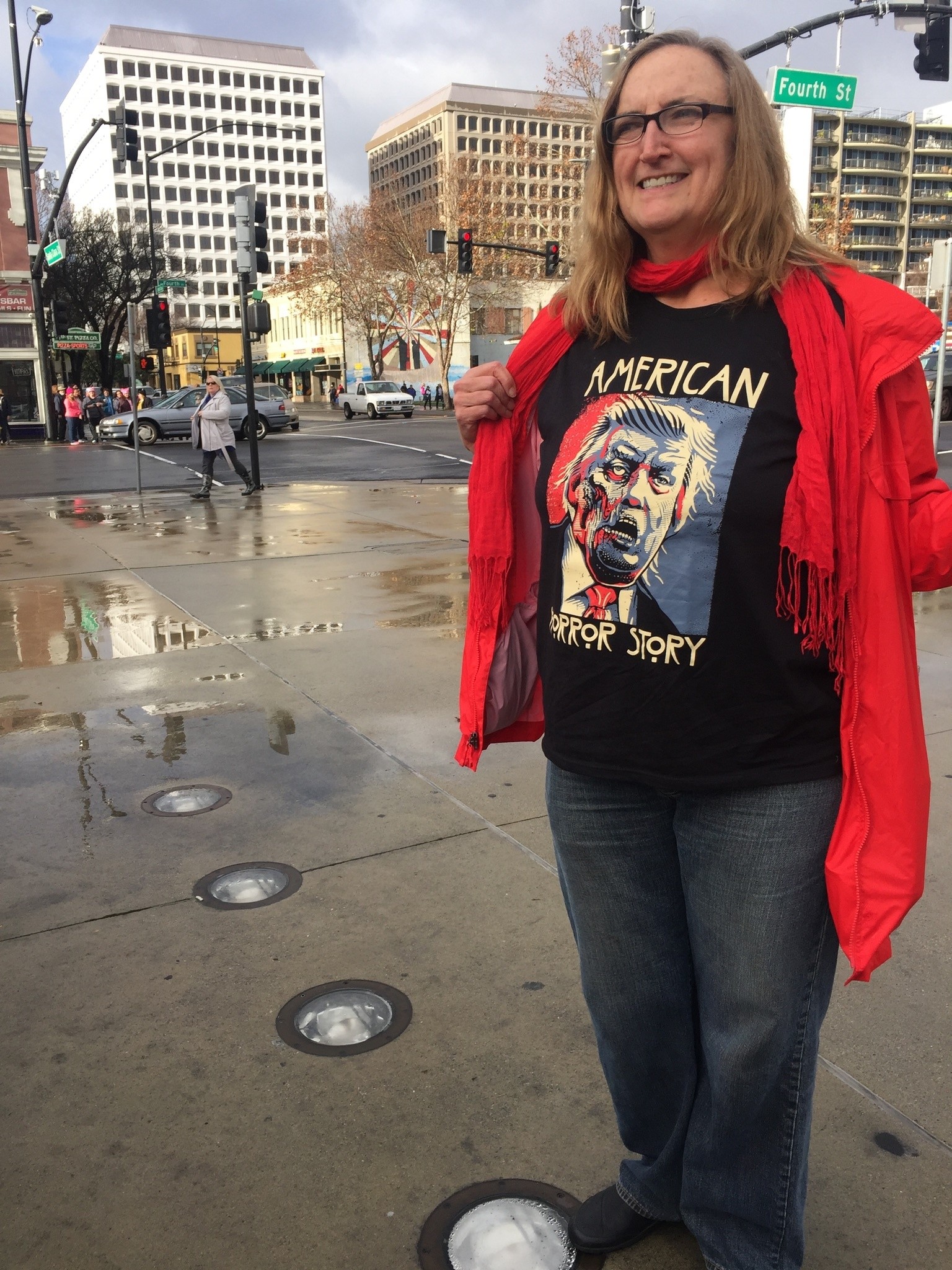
[
  {"x1": 152, "y1": 296, "x2": 172, "y2": 347},
  {"x1": 139, "y1": 357, "x2": 154, "y2": 369},
  {"x1": 457, "y1": 228, "x2": 473, "y2": 274},
  {"x1": 546, "y1": 240, "x2": 559, "y2": 277}
]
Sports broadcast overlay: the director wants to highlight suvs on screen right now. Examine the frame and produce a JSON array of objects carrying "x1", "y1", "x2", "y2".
[{"x1": 918, "y1": 347, "x2": 952, "y2": 421}]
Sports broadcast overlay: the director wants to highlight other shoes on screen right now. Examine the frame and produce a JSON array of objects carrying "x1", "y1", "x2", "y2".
[
  {"x1": 70, "y1": 441, "x2": 80, "y2": 445},
  {"x1": 91, "y1": 439, "x2": 97, "y2": 443},
  {"x1": 103, "y1": 439, "x2": 107, "y2": 442},
  {"x1": 59, "y1": 439, "x2": 65, "y2": 443},
  {"x1": 568, "y1": 1179, "x2": 665, "y2": 1254},
  {"x1": 78, "y1": 438, "x2": 86, "y2": 442}
]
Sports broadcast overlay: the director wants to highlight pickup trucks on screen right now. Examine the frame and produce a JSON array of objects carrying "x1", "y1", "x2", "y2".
[{"x1": 338, "y1": 380, "x2": 415, "y2": 419}]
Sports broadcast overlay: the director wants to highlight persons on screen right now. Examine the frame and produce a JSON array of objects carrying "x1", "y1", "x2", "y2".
[
  {"x1": 556, "y1": 397, "x2": 716, "y2": 636},
  {"x1": 189, "y1": 375, "x2": 257, "y2": 498},
  {"x1": 327, "y1": 382, "x2": 447, "y2": 411},
  {"x1": 193, "y1": 392, "x2": 203, "y2": 406},
  {"x1": 121, "y1": 387, "x2": 133, "y2": 411},
  {"x1": 71, "y1": 384, "x2": 88, "y2": 441},
  {"x1": 64, "y1": 387, "x2": 81, "y2": 446},
  {"x1": 102, "y1": 388, "x2": 118, "y2": 418},
  {"x1": 113, "y1": 390, "x2": 132, "y2": 414},
  {"x1": 82, "y1": 387, "x2": 108, "y2": 443},
  {"x1": 73, "y1": 389, "x2": 86, "y2": 443},
  {"x1": 136, "y1": 388, "x2": 153, "y2": 411},
  {"x1": 98, "y1": 387, "x2": 104, "y2": 397},
  {"x1": 450, "y1": 29, "x2": 952, "y2": 1265},
  {"x1": 53, "y1": 386, "x2": 68, "y2": 444},
  {"x1": 0, "y1": 387, "x2": 13, "y2": 444}
]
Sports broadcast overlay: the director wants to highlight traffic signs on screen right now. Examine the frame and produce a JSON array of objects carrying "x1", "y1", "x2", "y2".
[
  {"x1": 157, "y1": 278, "x2": 186, "y2": 287},
  {"x1": 766, "y1": 66, "x2": 858, "y2": 113},
  {"x1": 50, "y1": 331, "x2": 102, "y2": 350},
  {"x1": 155, "y1": 283, "x2": 164, "y2": 295}
]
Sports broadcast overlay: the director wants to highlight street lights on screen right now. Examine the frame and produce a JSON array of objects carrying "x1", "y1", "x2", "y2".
[
  {"x1": 144, "y1": 121, "x2": 303, "y2": 396},
  {"x1": 200, "y1": 313, "x2": 221, "y2": 384},
  {"x1": 5, "y1": 1, "x2": 65, "y2": 444},
  {"x1": 190, "y1": 302, "x2": 221, "y2": 370}
]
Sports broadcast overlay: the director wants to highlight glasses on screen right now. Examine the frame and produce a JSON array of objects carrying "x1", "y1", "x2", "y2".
[
  {"x1": 205, "y1": 381, "x2": 216, "y2": 386},
  {"x1": 601, "y1": 99, "x2": 739, "y2": 146}
]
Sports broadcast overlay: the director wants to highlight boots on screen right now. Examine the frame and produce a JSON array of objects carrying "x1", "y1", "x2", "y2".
[
  {"x1": 189, "y1": 474, "x2": 213, "y2": 499},
  {"x1": 235, "y1": 468, "x2": 257, "y2": 496}
]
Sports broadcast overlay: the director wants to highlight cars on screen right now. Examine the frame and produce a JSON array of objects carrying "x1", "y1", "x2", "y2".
[
  {"x1": 97, "y1": 391, "x2": 268, "y2": 446},
  {"x1": 232, "y1": 382, "x2": 300, "y2": 429},
  {"x1": 83, "y1": 386, "x2": 180, "y2": 407}
]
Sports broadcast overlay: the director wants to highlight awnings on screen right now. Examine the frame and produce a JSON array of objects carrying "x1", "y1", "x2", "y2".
[{"x1": 233, "y1": 357, "x2": 325, "y2": 374}]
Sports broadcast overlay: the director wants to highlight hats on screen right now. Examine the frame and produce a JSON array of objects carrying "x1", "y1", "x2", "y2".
[
  {"x1": 121, "y1": 387, "x2": 130, "y2": 396},
  {"x1": 57, "y1": 386, "x2": 67, "y2": 391},
  {"x1": 138, "y1": 389, "x2": 146, "y2": 397},
  {"x1": 86, "y1": 387, "x2": 95, "y2": 392},
  {"x1": 65, "y1": 387, "x2": 73, "y2": 395}
]
